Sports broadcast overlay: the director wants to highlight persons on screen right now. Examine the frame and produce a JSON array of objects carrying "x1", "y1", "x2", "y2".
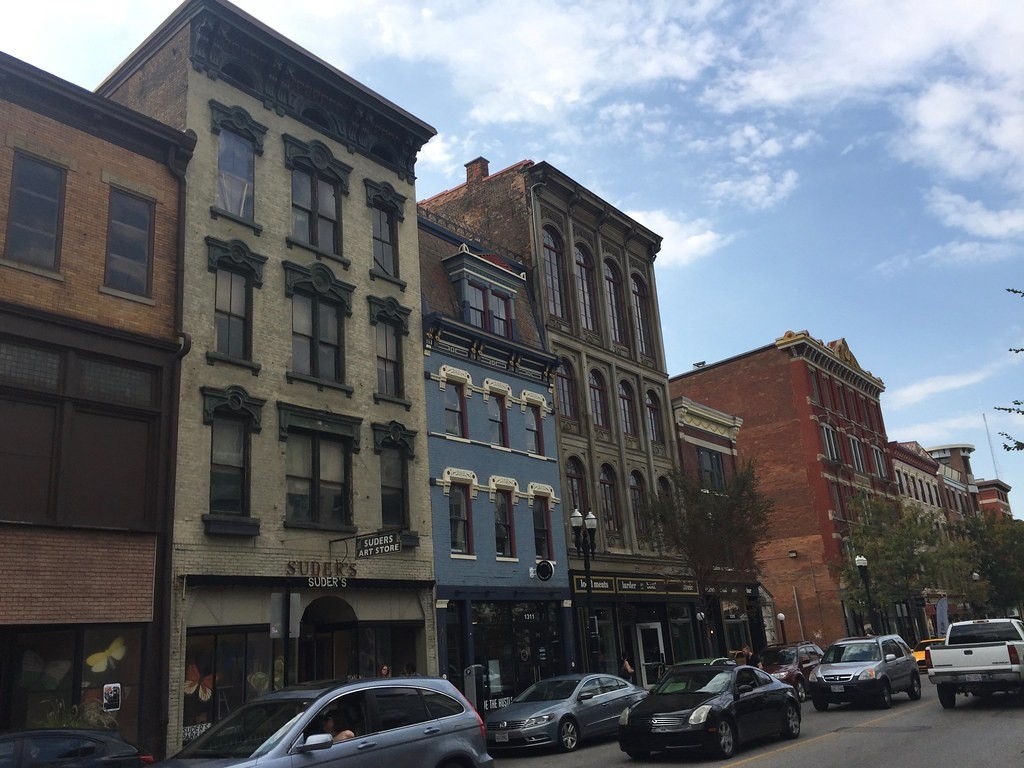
[
  {"x1": 742, "y1": 645, "x2": 763, "y2": 670},
  {"x1": 620, "y1": 651, "x2": 634, "y2": 683},
  {"x1": 320, "y1": 712, "x2": 355, "y2": 742},
  {"x1": 377, "y1": 664, "x2": 392, "y2": 677}
]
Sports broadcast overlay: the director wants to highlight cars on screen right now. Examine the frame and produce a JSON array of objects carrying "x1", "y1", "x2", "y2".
[
  {"x1": 155, "y1": 674, "x2": 496, "y2": 768},
  {"x1": 649, "y1": 657, "x2": 737, "y2": 694},
  {"x1": 911, "y1": 638, "x2": 948, "y2": 672},
  {"x1": 616, "y1": 665, "x2": 803, "y2": 760},
  {"x1": 0, "y1": 728, "x2": 160, "y2": 768},
  {"x1": 483, "y1": 670, "x2": 649, "y2": 753}
]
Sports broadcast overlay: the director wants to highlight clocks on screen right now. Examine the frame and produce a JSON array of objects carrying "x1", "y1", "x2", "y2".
[{"x1": 536, "y1": 561, "x2": 554, "y2": 581}]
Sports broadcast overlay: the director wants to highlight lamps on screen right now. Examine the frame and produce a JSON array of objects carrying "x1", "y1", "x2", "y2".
[
  {"x1": 777, "y1": 611, "x2": 786, "y2": 622},
  {"x1": 697, "y1": 612, "x2": 705, "y2": 622}
]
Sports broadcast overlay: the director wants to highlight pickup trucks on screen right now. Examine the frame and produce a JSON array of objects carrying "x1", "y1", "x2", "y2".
[{"x1": 924, "y1": 618, "x2": 1023, "y2": 708}]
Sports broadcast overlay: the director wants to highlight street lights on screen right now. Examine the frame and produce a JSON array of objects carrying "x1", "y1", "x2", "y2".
[
  {"x1": 854, "y1": 555, "x2": 879, "y2": 634},
  {"x1": 572, "y1": 505, "x2": 600, "y2": 672}
]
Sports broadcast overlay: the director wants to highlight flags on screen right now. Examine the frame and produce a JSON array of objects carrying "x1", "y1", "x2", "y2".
[{"x1": 936, "y1": 597, "x2": 950, "y2": 638}]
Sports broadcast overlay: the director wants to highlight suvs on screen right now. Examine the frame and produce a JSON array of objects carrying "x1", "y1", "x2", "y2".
[
  {"x1": 749, "y1": 641, "x2": 824, "y2": 702},
  {"x1": 807, "y1": 633, "x2": 921, "y2": 709}
]
